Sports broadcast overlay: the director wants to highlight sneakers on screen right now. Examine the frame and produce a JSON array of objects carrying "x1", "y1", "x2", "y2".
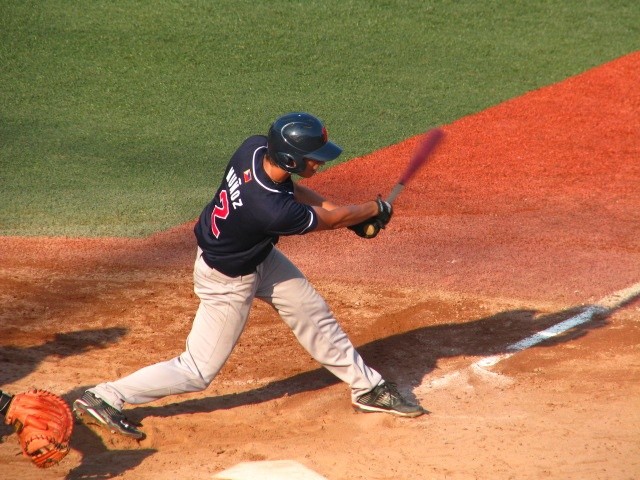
[
  {"x1": 74, "y1": 390, "x2": 144, "y2": 439},
  {"x1": 351, "y1": 381, "x2": 423, "y2": 417}
]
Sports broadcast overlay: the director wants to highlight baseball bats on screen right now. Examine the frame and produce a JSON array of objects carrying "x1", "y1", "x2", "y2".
[{"x1": 362, "y1": 127, "x2": 443, "y2": 238}]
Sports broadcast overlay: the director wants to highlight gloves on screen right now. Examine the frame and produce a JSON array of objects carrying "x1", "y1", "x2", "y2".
[{"x1": 347, "y1": 198, "x2": 393, "y2": 238}]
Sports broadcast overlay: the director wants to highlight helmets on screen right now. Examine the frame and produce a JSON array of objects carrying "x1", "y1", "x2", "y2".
[{"x1": 268, "y1": 113, "x2": 343, "y2": 175}]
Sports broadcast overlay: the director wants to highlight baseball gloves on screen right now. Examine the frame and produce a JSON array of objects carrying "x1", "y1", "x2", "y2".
[{"x1": 7, "y1": 389, "x2": 74, "y2": 469}]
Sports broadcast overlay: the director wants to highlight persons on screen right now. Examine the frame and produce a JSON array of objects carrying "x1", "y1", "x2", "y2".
[
  {"x1": 73, "y1": 109, "x2": 425, "y2": 440},
  {"x1": 0, "y1": 394, "x2": 61, "y2": 430}
]
